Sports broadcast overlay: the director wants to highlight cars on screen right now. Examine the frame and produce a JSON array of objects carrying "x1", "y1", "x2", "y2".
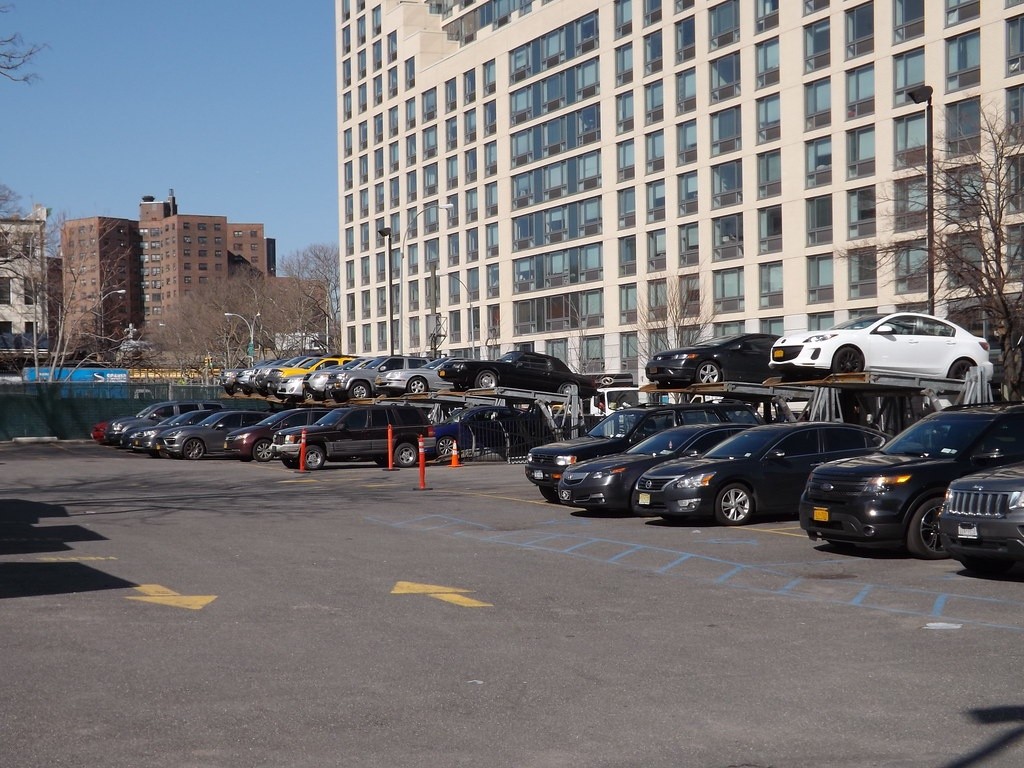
[
  {"x1": 267, "y1": 403, "x2": 436, "y2": 471},
  {"x1": 92, "y1": 400, "x2": 227, "y2": 458},
  {"x1": 373, "y1": 356, "x2": 476, "y2": 395},
  {"x1": 427, "y1": 405, "x2": 545, "y2": 459},
  {"x1": 769, "y1": 312, "x2": 994, "y2": 382},
  {"x1": 218, "y1": 353, "x2": 372, "y2": 402},
  {"x1": 645, "y1": 333, "x2": 782, "y2": 385},
  {"x1": 558, "y1": 424, "x2": 758, "y2": 511},
  {"x1": 154, "y1": 411, "x2": 275, "y2": 460},
  {"x1": 223, "y1": 407, "x2": 334, "y2": 462},
  {"x1": 937, "y1": 461, "x2": 1024, "y2": 576},
  {"x1": 438, "y1": 351, "x2": 597, "y2": 404},
  {"x1": 632, "y1": 421, "x2": 924, "y2": 526}
]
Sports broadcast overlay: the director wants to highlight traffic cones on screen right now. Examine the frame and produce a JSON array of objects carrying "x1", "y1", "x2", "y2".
[{"x1": 448, "y1": 440, "x2": 462, "y2": 467}]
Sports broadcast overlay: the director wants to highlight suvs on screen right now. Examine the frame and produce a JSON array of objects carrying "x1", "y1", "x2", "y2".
[
  {"x1": 525, "y1": 398, "x2": 767, "y2": 502},
  {"x1": 325, "y1": 354, "x2": 430, "y2": 399},
  {"x1": 799, "y1": 400, "x2": 1024, "y2": 559}
]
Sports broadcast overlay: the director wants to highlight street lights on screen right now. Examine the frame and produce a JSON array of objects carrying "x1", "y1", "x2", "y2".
[
  {"x1": 224, "y1": 313, "x2": 261, "y2": 368},
  {"x1": 377, "y1": 227, "x2": 394, "y2": 356},
  {"x1": 400, "y1": 203, "x2": 454, "y2": 355},
  {"x1": 437, "y1": 272, "x2": 475, "y2": 359},
  {"x1": 95, "y1": 289, "x2": 127, "y2": 344},
  {"x1": 908, "y1": 86, "x2": 935, "y2": 333}
]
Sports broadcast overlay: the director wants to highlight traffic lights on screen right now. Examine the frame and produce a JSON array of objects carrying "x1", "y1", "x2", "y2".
[
  {"x1": 209, "y1": 357, "x2": 212, "y2": 366},
  {"x1": 205, "y1": 357, "x2": 208, "y2": 366}
]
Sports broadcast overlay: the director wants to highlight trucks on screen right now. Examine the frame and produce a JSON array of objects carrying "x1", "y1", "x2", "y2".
[
  {"x1": 275, "y1": 332, "x2": 321, "y2": 351},
  {"x1": 551, "y1": 387, "x2": 651, "y2": 437}
]
